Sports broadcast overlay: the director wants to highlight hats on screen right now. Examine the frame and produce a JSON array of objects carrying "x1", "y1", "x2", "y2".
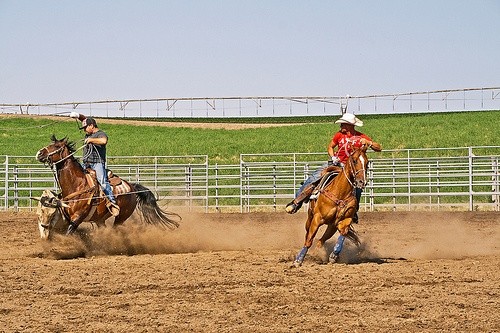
[
  {"x1": 79, "y1": 118, "x2": 97, "y2": 129},
  {"x1": 335, "y1": 113, "x2": 363, "y2": 127}
]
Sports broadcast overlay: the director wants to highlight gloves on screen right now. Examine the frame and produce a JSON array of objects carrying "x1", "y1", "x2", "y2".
[
  {"x1": 331, "y1": 156, "x2": 341, "y2": 166},
  {"x1": 70, "y1": 112, "x2": 80, "y2": 119}
]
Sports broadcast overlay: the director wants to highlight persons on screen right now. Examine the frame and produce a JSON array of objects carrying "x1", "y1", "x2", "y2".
[
  {"x1": 69, "y1": 112, "x2": 119, "y2": 217},
  {"x1": 284, "y1": 113, "x2": 381, "y2": 224}
]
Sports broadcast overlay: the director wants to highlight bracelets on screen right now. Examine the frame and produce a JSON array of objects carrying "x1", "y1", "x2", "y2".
[{"x1": 370, "y1": 140, "x2": 372, "y2": 147}]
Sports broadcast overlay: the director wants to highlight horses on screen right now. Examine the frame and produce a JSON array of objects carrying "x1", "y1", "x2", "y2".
[
  {"x1": 35, "y1": 131, "x2": 181, "y2": 235},
  {"x1": 290, "y1": 141, "x2": 368, "y2": 267}
]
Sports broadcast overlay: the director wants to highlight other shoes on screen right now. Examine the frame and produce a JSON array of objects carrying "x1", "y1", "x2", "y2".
[
  {"x1": 353, "y1": 211, "x2": 359, "y2": 222},
  {"x1": 109, "y1": 204, "x2": 119, "y2": 216},
  {"x1": 285, "y1": 202, "x2": 297, "y2": 214}
]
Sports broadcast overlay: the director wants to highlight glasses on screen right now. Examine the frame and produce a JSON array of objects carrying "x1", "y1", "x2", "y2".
[{"x1": 340, "y1": 123, "x2": 349, "y2": 128}]
